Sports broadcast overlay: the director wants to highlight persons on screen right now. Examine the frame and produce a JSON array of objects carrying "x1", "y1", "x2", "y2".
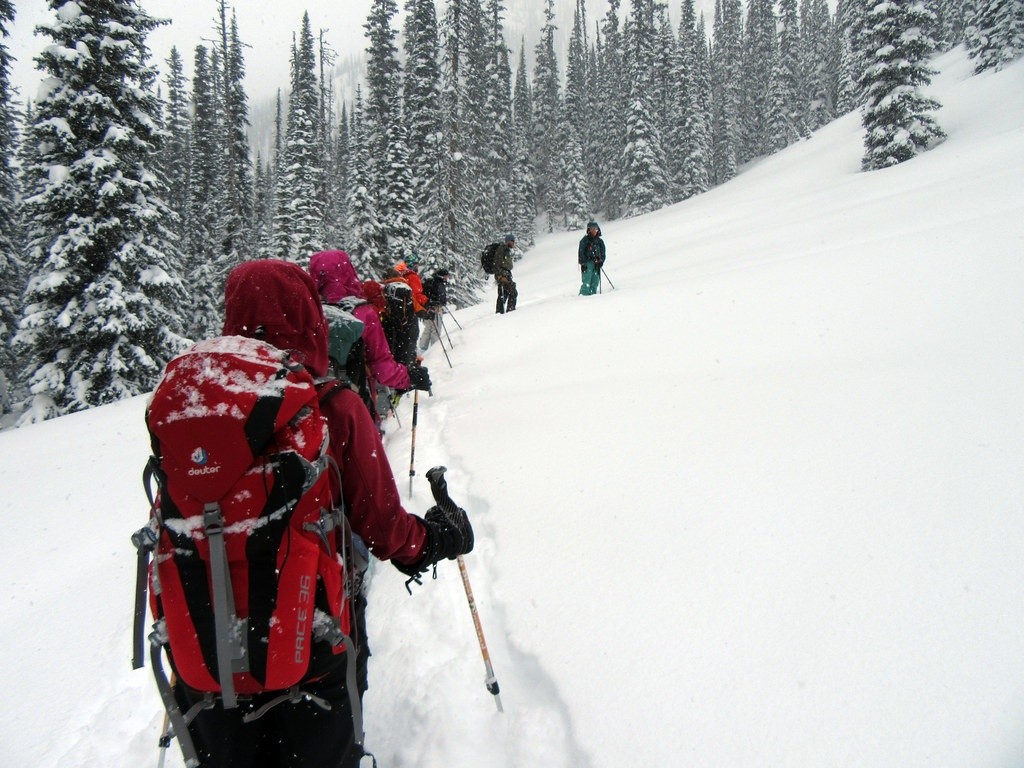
[
  {"x1": 578, "y1": 222, "x2": 606, "y2": 295},
  {"x1": 362, "y1": 261, "x2": 449, "y2": 366},
  {"x1": 493, "y1": 234, "x2": 518, "y2": 314},
  {"x1": 306, "y1": 250, "x2": 430, "y2": 435},
  {"x1": 147, "y1": 257, "x2": 475, "y2": 768}
]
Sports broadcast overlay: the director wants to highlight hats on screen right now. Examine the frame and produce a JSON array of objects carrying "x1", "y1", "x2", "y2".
[
  {"x1": 505, "y1": 234, "x2": 515, "y2": 243},
  {"x1": 404, "y1": 254, "x2": 419, "y2": 269}
]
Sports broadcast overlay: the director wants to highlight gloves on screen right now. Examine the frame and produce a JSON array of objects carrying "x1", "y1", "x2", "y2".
[
  {"x1": 499, "y1": 277, "x2": 511, "y2": 283},
  {"x1": 395, "y1": 362, "x2": 432, "y2": 397},
  {"x1": 595, "y1": 261, "x2": 603, "y2": 270},
  {"x1": 417, "y1": 310, "x2": 430, "y2": 320},
  {"x1": 582, "y1": 265, "x2": 587, "y2": 272},
  {"x1": 391, "y1": 504, "x2": 474, "y2": 578}
]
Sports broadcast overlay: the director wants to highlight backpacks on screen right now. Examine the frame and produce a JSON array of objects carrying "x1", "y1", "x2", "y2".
[
  {"x1": 144, "y1": 335, "x2": 351, "y2": 697},
  {"x1": 322, "y1": 295, "x2": 369, "y2": 405},
  {"x1": 382, "y1": 282, "x2": 414, "y2": 334},
  {"x1": 423, "y1": 278, "x2": 439, "y2": 303},
  {"x1": 482, "y1": 242, "x2": 507, "y2": 274}
]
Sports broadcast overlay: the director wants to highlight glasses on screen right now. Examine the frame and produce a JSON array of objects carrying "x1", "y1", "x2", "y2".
[{"x1": 511, "y1": 240, "x2": 515, "y2": 243}]
